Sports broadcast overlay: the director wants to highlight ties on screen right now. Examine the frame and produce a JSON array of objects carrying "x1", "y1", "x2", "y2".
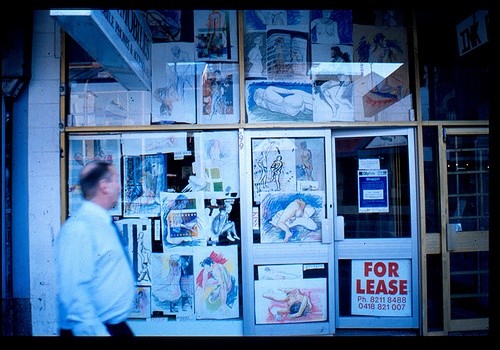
[{"x1": 111, "y1": 215, "x2": 138, "y2": 282}]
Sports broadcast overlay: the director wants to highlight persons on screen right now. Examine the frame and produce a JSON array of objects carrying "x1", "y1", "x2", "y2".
[{"x1": 54, "y1": 159, "x2": 139, "y2": 337}]
